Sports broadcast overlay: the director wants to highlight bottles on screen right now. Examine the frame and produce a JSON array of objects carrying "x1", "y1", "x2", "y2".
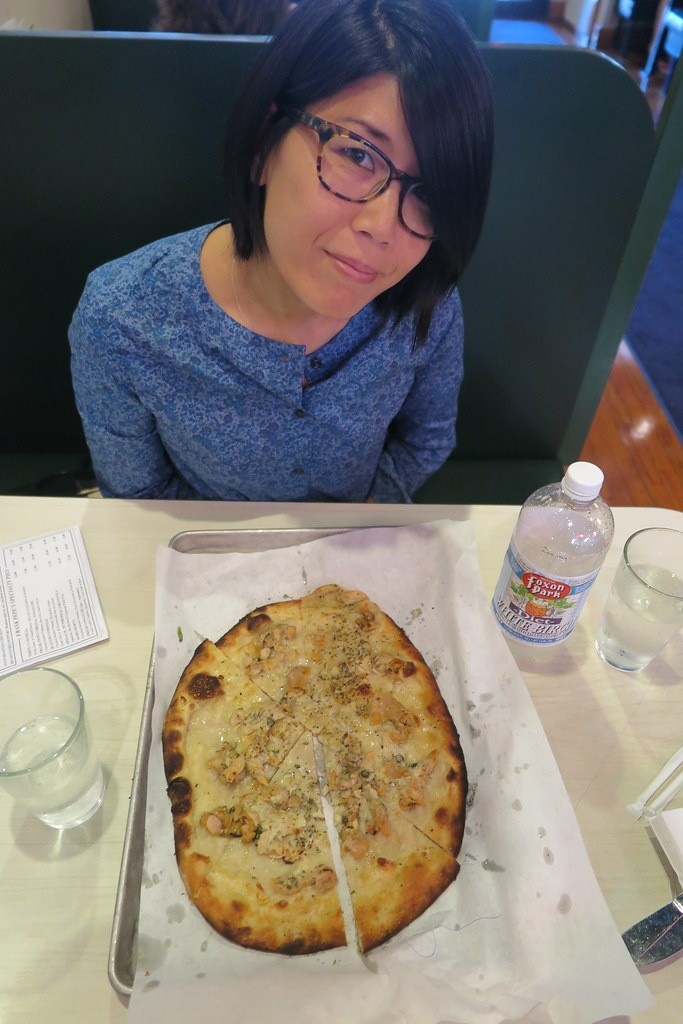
[{"x1": 489, "y1": 460, "x2": 616, "y2": 648}]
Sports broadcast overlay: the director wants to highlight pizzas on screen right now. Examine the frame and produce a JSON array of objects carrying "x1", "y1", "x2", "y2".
[{"x1": 161, "y1": 584, "x2": 470, "y2": 955}]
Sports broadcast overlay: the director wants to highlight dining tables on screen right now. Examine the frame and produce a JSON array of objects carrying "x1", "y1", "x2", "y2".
[{"x1": 0, "y1": 493, "x2": 683, "y2": 1024}]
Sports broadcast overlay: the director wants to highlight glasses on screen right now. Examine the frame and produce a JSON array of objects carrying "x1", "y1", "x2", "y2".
[{"x1": 274, "y1": 107, "x2": 443, "y2": 241}]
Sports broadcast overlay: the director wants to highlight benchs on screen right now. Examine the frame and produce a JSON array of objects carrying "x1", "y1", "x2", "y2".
[{"x1": 1, "y1": 31, "x2": 664, "y2": 504}]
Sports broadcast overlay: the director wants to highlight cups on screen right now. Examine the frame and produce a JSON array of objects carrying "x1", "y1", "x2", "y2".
[
  {"x1": 593, "y1": 527, "x2": 683, "y2": 674},
  {"x1": 0, "y1": 666, "x2": 107, "y2": 830}
]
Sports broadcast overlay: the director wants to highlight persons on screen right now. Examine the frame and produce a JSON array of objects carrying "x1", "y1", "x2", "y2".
[{"x1": 69, "y1": 0, "x2": 495, "y2": 503}]
